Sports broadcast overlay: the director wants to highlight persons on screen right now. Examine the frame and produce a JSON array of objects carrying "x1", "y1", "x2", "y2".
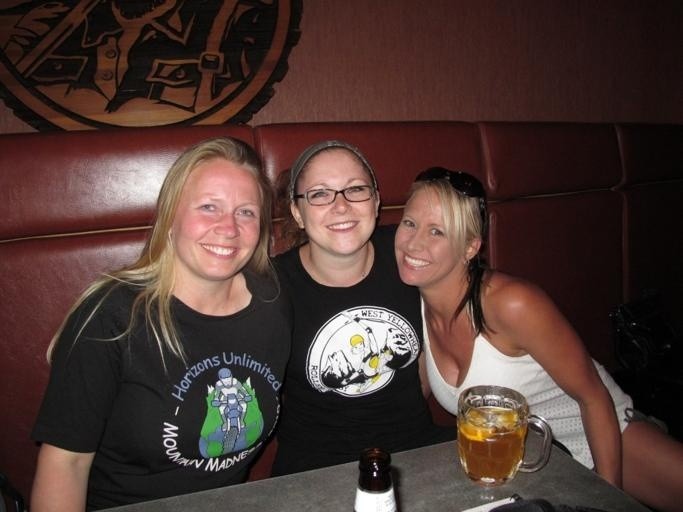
[
  {"x1": 391, "y1": 150, "x2": 682, "y2": 510},
  {"x1": 259, "y1": 140, "x2": 446, "y2": 483},
  {"x1": 31, "y1": 134, "x2": 293, "y2": 512}
]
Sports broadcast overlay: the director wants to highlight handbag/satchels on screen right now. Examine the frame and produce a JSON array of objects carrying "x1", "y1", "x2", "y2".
[{"x1": 609, "y1": 297, "x2": 682, "y2": 387}]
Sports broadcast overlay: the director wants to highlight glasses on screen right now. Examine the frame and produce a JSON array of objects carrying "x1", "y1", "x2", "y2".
[
  {"x1": 292, "y1": 184, "x2": 374, "y2": 204},
  {"x1": 413, "y1": 165, "x2": 488, "y2": 244}
]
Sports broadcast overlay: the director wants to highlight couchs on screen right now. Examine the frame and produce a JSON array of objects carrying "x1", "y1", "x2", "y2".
[{"x1": 0, "y1": 119, "x2": 680, "y2": 509}]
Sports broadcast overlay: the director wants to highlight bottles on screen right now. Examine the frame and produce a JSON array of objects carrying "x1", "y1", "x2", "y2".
[{"x1": 352, "y1": 447, "x2": 398, "y2": 512}]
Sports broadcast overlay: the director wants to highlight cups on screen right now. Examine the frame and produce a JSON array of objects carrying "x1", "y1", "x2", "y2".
[{"x1": 457, "y1": 385, "x2": 554, "y2": 487}]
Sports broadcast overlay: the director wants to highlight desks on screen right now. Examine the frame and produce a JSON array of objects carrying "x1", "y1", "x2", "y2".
[{"x1": 105, "y1": 423, "x2": 656, "y2": 512}]
